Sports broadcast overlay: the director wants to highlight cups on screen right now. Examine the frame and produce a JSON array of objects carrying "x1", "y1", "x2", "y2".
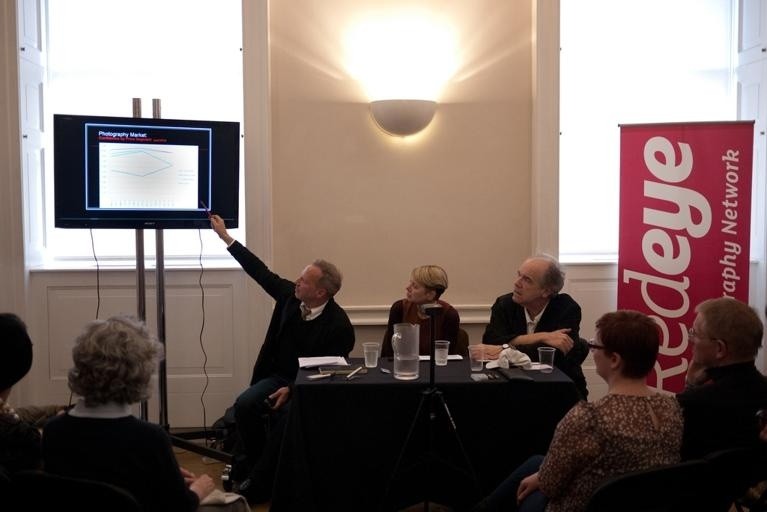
[
  {"x1": 362, "y1": 342, "x2": 379, "y2": 368},
  {"x1": 467, "y1": 344, "x2": 484, "y2": 371},
  {"x1": 537, "y1": 347, "x2": 554, "y2": 374},
  {"x1": 434, "y1": 340, "x2": 449, "y2": 367}
]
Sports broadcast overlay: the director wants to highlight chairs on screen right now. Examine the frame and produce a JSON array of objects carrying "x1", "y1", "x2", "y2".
[
  {"x1": 10, "y1": 469, "x2": 138, "y2": 512},
  {"x1": 585, "y1": 458, "x2": 712, "y2": 512},
  {"x1": 567, "y1": 338, "x2": 590, "y2": 402},
  {"x1": 704, "y1": 435, "x2": 767, "y2": 512}
]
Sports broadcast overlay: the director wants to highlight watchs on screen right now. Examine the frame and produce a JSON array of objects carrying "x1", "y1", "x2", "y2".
[{"x1": 502, "y1": 343, "x2": 509, "y2": 350}]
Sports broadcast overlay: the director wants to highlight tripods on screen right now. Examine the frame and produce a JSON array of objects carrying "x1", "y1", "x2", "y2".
[{"x1": 392, "y1": 390, "x2": 484, "y2": 512}]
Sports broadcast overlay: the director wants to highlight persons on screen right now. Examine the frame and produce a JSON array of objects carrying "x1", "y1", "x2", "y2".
[
  {"x1": 209, "y1": 214, "x2": 354, "y2": 444},
  {"x1": 467, "y1": 253, "x2": 581, "y2": 370},
  {"x1": 477, "y1": 311, "x2": 685, "y2": 511},
  {"x1": 0, "y1": 311, "x2": 43, "y2": 512},
  {"x1": 676, "y1": 297, "x2": 767, "y2": 460},
  {"x1": 380, "y1": 265, "x2": 460, "y2": 358},
  {"x1": 41, "y1": 317, "x2": 250, "y2": 512}
]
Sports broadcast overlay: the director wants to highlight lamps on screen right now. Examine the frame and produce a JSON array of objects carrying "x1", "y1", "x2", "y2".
[{"x1": 366, "y1": 98, "x2": 437, "y2": 137}]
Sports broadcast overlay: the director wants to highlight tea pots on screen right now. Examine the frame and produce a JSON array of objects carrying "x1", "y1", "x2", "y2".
[{"x1": 390, "y1": 323, "x2": 420, "y2": 381}]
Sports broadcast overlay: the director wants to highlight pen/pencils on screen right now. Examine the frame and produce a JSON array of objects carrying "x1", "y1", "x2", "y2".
[
  {"x1": 200, "y1": 200, "x2": 213, "y2": 219},
  {"x1": 346, "y1": 366, "x2": 362, "y2": 378},
  {"x1": 485, "y1": 371, "x2": 500, "y2": 380}
]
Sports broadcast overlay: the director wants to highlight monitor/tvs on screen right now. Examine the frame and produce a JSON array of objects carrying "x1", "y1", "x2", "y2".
[{"x1": 53, "y1": 113, "x2": 240, "y2": 231}]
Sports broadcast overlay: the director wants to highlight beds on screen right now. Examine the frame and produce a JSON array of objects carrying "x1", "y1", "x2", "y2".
[{"x1": 455, "y1": 330, "x2": 469, "y2": 358}]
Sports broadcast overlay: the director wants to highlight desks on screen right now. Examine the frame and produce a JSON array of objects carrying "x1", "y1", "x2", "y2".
[{"x1": 277, "y1": 357, "x2": 576, "y2": 512}]
